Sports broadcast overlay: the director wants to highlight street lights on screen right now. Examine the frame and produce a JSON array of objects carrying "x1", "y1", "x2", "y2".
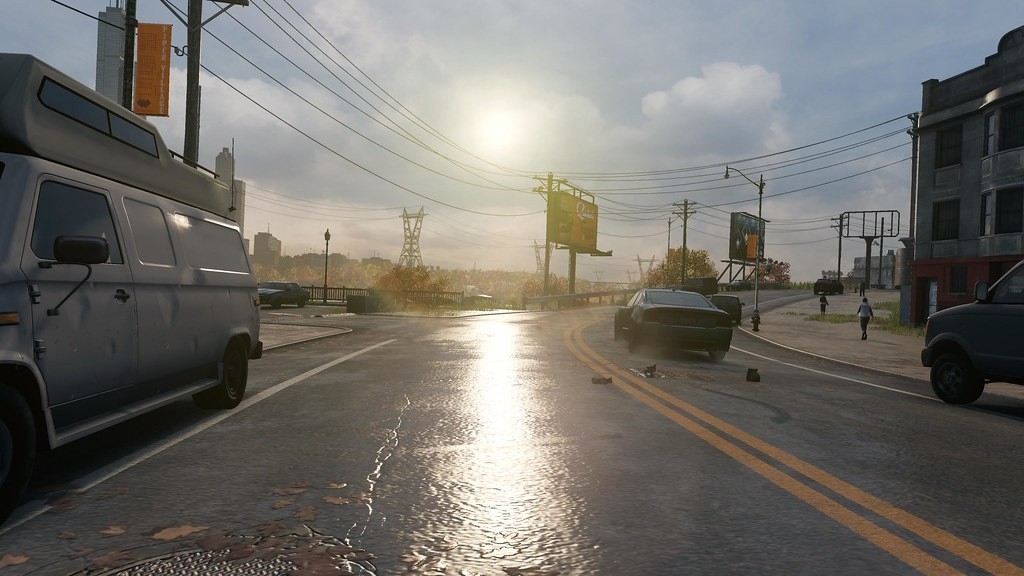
[
  {"x1": 322, "y1": 228, "x2": 331, "y2": 306},
  {"x1": 723, "y1": 164, "x2": 765, "y2": 332}
]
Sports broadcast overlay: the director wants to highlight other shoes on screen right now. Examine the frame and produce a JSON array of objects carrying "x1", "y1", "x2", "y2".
[
  {"x1": 861, "y1": 337, "x2": 864, "y2": 340},
  {"x1": 864, "y1": 335, "x2": 867, "y2": 340}
]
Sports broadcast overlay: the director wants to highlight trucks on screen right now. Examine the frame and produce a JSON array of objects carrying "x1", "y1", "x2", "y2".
[{"x1": 1, "y1": 53, "x2": 264, "y2": 534}]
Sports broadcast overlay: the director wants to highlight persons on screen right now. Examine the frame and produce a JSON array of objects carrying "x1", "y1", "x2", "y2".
[
  {"x1": 820, "y1": 293, "x2": 828, "y2": 315},
  {"x1": 857, "y1": 298, "x2": 873, "y2": 340},
  {"x1": 860, "y1": 280, "x2": 865, "y2": 296}
]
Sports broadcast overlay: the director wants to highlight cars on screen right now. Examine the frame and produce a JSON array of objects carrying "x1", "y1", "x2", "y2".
[
  {"x1": 813, "y1": 279, "x2": 844, "y2": 296},
  {"x1": 614, "y1": 288, "x2": 732, "y2": 363},
  {"x1": 711, "y1": 294, "x2": 746, "y2": 324},
  {"x1": 257, "y1": 281, "x2": 310, "y2": 310}
]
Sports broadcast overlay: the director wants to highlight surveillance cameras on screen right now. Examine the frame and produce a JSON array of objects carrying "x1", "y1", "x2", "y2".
[{"x1": 724, "y1": 174, "x2": 729, "y2": 179}]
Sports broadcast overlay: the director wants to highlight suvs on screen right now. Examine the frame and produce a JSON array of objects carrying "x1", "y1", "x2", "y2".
[
  {"x1": 920, "y1": 257, "x2": 1023, "y2": 405},
  {"x1": 678, "y1": 276, "x2": 719, "y2": 297}
]
